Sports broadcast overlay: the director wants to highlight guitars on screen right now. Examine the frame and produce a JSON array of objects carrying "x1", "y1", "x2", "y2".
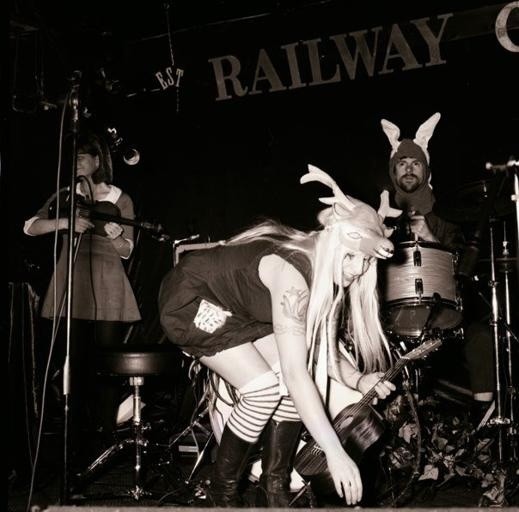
[{"x1": 292, "y1": 328, "x2": 444, "y2": 489}]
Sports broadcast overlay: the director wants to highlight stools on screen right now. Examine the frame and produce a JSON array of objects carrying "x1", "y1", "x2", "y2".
[{"x1": 67, "y1": 347, "x2": 186, "y2": 500}]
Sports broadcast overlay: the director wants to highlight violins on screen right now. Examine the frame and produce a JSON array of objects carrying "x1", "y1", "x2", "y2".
[{"x1": 48, "y1": 189, "x2": 162, "y2": 237}]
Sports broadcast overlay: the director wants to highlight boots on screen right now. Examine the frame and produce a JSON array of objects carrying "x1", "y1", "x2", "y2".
[
  {"x1": 259, "y1": 417, "x2": 304, "y2": 507},
  {"x1": 209, "y1": 423, "x2": 260, "y2": 507}
]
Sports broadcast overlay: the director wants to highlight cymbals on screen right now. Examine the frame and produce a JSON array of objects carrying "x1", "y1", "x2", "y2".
[{"x1": 471, "y1": 257, "x2": 518, "y2": 279}]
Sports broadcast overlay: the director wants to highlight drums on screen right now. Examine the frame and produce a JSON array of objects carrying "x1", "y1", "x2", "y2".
[{"x1": 377, "y1": 241, "x2": 464, "y2": 339}]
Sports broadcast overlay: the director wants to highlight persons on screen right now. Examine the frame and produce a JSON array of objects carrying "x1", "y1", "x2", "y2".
[
  {"x1": 21, "y1": 133, "x2": 143, "y2": 460},
  {"x1": 155, "y1": 162, "x2": 404, "y2": 507},
  {"x1": 374, "y1": 108, "x2": 479, "y2": 283}
]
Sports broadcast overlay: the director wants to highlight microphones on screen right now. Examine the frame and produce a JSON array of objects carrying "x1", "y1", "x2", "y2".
[{"x1": 101, "y1": 116, "x2": 140, "y2": 165}]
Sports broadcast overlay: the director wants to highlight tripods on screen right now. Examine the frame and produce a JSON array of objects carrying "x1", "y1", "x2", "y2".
[{"x1": 385, "y1": 170, "x2": 519, "y2": 501}]
